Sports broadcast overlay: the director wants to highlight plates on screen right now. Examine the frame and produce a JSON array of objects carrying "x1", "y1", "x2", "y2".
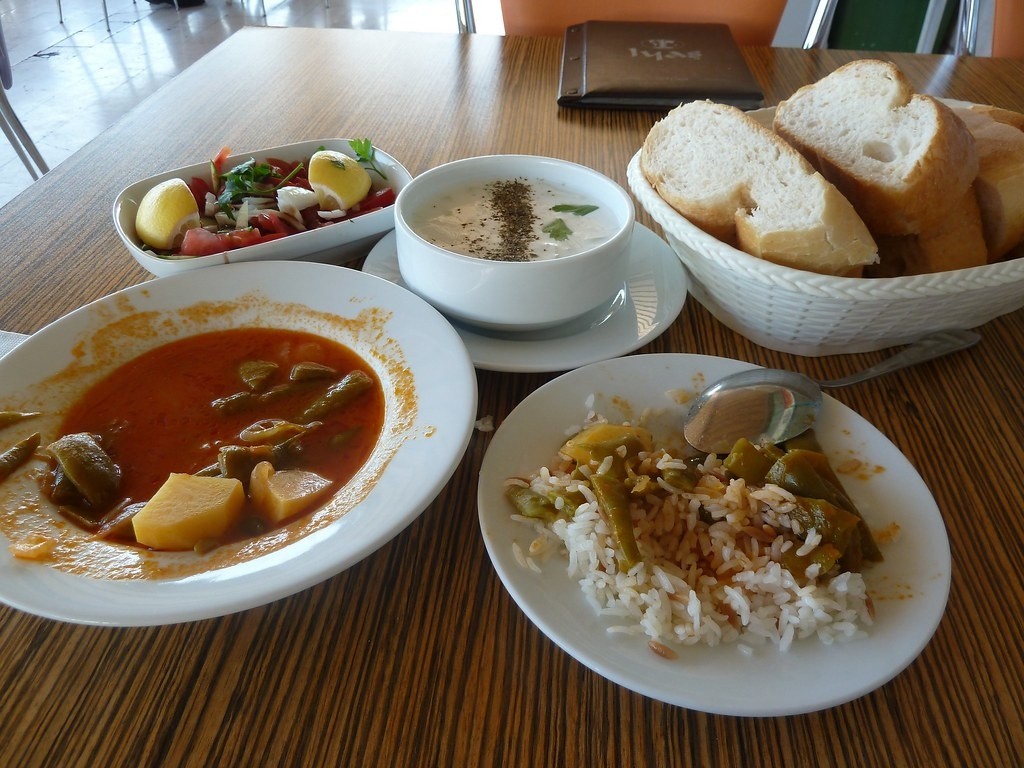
[
  {"x1": 358, "y1": 220, "x2": 688, "y2": 375},
  {"x1": 478, "y1": 352, "x2": 951, "y2": 717},
  {"x1": 1, "y1": 258, "x2": 478, "y2": 627}
]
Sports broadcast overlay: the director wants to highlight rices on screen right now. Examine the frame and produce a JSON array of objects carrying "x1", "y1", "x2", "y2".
[{"x1": 504, "y1": 446, "x2": 875, "y2": 661}]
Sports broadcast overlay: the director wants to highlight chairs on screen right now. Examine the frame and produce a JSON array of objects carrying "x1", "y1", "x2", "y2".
[
  {"x1": 952, "y1": 1, "x2": 1024, "y2": 61},
  {"x1": 457, "y1": 0, "x2": 838, "y2": 74}
]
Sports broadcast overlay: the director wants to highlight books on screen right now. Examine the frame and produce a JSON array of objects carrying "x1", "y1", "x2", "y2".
[{"x1": 555, "y1": 18, "x2": 766, "y2": 116}]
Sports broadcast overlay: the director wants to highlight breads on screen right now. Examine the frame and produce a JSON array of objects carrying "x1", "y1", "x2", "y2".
[{"x1": 641, "y1": 56, "x2": 1024, "y2": 279}]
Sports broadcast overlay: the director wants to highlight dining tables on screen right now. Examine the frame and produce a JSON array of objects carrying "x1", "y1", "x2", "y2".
[{"x1": 1, "y1": 26, "x2": 1024, "y2": 768}]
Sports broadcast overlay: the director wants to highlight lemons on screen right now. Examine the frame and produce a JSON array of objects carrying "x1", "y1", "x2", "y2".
[
  {"x1": 134, "y1": 177, "x2": 201, "y2": 254},
  {"x1": 308, "y1": 149, "x2": 372, "y2": 212}
]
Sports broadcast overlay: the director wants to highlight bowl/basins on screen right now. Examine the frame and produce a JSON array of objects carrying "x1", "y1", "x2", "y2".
[
  {"x1": 112, "y1": 137, "x2": 413, "y2": 277},
  {"x1": 626, "y1": 97, "x2": 1024, "y2": 357},
  {"x1": 394, "y1": 154, "x2": 636, "y2": 330}
]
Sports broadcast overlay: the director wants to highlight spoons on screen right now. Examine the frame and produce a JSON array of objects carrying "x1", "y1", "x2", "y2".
[{"x1": 684, "y1": 329, "x2": 983, "y2": 453}]
